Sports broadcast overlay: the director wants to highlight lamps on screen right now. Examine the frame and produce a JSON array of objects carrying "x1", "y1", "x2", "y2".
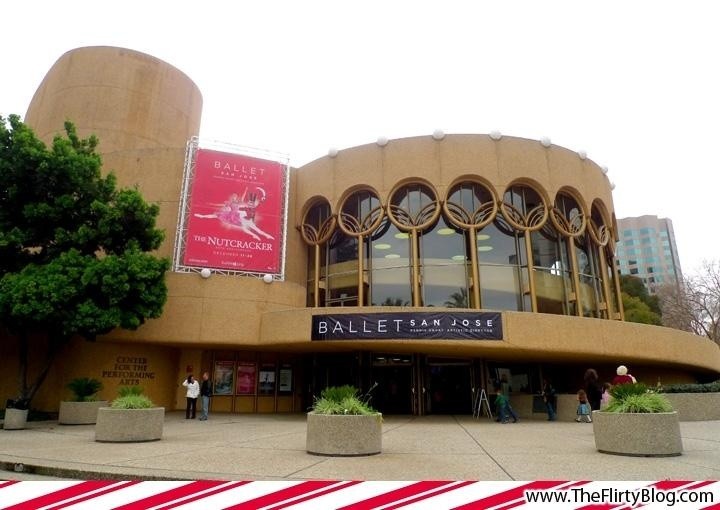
[
  {"x1": 201, "y1": 268, "x2": 212, "y2": 279},
  {"x1": 263, "y1": 273, "x2": 274, "y2": 284}
]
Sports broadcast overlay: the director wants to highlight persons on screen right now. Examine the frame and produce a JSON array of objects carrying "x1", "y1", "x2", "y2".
[
  {"x1": 575, "y1": 390, "x2": 592, "y2": 423},
  {"x1": 611, "y1": 365, "x2": 633, "y2": 386},
  {"x1": 541, "y1": 377, "x2": 556, "y2": 420},
  {"x1": 602, "y1": 383, "x2": 611, "y2": 404},
  {"x1": 494, "y1": 390, "x2": 507, "y2": 423},
  {"x1": 183, "y1": 375, "x2": 200, "y2": 419},
  {"x1": 239, "y1": 187, "x2": 275, "y2": 239},
  {"x1": 496, "y1": 374, "x2": 520, "y2": 423},
  {"x1": 582, "y1": 368, "x2": 601, "y2": 409},
  {"x1": 626, "y1": 373, "x2": 637, "y2": 384},
  {"x1": 194, "y1": 186, "x2": 261, "y2": 241},
  {"x1": 200, "y1": 370, "x2": 212, "y2": 421}
]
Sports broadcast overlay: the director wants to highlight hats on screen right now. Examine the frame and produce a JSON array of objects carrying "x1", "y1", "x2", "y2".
[{"x1": 617, "y1": 365, "x2": 628, "y2": 376}]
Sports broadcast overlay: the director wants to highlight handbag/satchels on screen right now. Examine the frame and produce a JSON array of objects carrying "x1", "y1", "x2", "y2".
[{"x1": 545, "y1": 384, "x2": 555, "y2": 396}]
